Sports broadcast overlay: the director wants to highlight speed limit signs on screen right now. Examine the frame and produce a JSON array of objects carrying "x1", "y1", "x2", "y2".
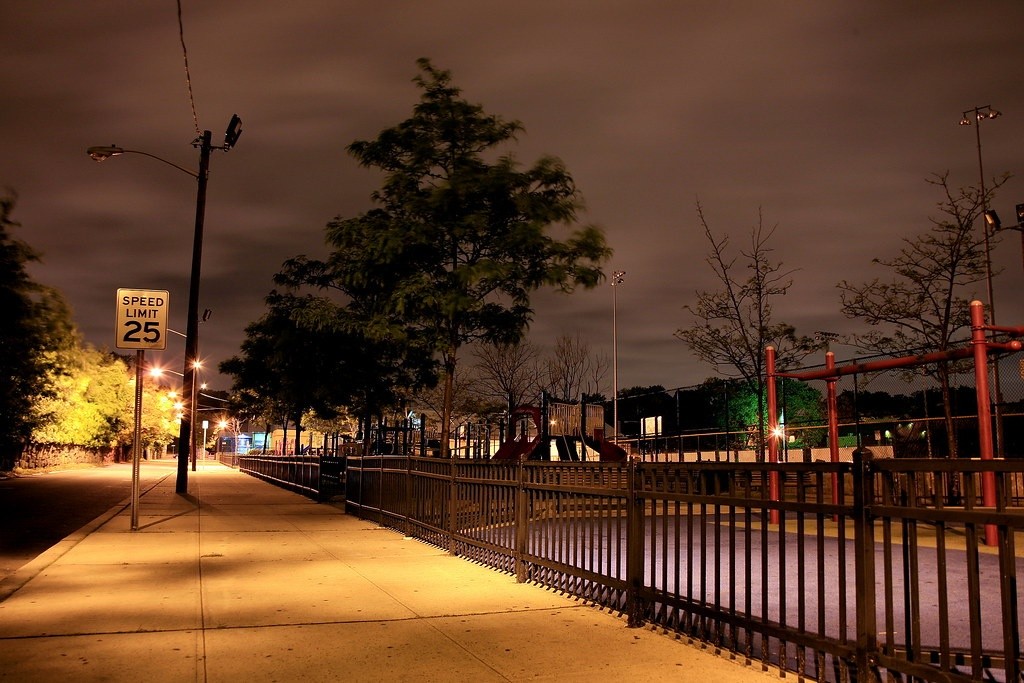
[{"x1": 114, "y1": 287, "x2": 170, "y2": 351}]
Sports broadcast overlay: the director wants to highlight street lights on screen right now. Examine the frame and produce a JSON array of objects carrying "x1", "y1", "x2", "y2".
[
  {"x1": 960, "y1": 105, "x2": 1004, "y2": 467},
  {"x1": 89, "y1": 142, "x2": 210, "y2": 496},
  {"x1": 611, "y1": 270, "x2": 626, "y2": 445}
]
